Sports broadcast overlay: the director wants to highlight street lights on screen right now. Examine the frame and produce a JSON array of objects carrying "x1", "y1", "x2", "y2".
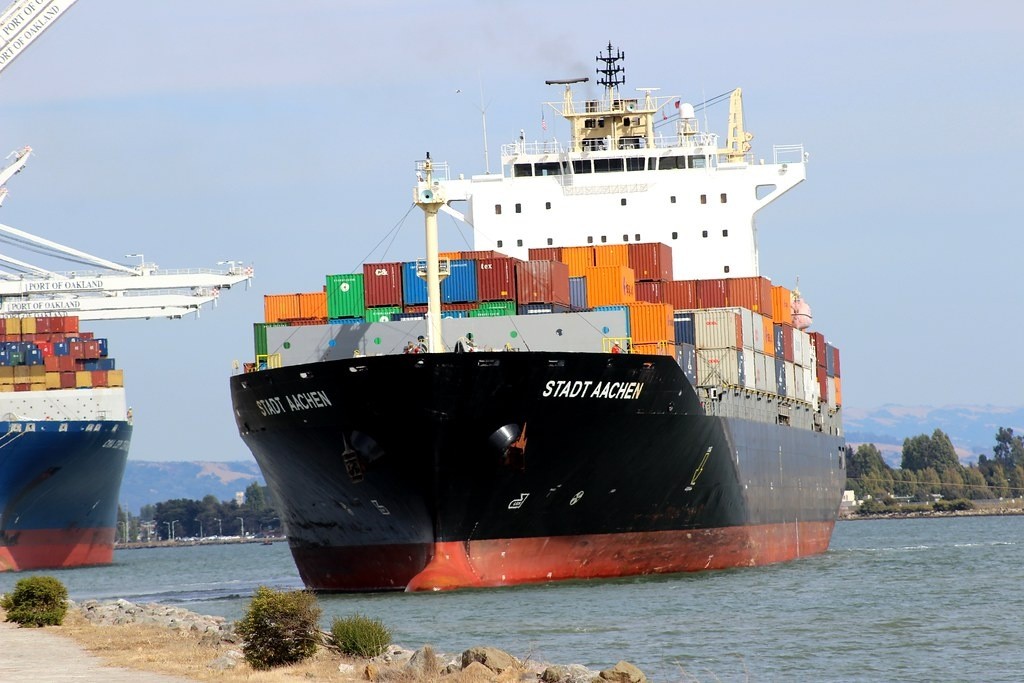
[
  {"x1": 163, "y1": 521, "x2": 171, "y2": 540},
  {"x1": 195, "y1": 519, "x2": 202, "y2": 538},
  {"x1": 236, "y1": 517, "x2": 244, "y2": 536},
  {"x1": 171, "y1": 519, "x2": 179, "y2": 540},
  {"x1": 214, "y1": 518, "x2": 222, "y2": 536}
]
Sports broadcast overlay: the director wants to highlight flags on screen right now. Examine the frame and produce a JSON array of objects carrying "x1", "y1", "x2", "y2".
[{"x1": 542, "y1": 111, "x2": 546, "y2": 131}]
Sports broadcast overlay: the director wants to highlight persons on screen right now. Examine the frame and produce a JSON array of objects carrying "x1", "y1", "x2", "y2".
[
  {"x1": 612, "y1": 341, "x2": 621, "y2": 353},
  {"x1": 465, "y1": 333, "x2": 474, "y2": 352},
  {"x1": 402, "y1": 335, "x2": 427, "y2": 353}
]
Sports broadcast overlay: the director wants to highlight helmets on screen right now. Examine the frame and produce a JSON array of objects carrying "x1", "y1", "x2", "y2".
[{"x1": 615, "y1": 341, "x2": 619, "y2": 344}]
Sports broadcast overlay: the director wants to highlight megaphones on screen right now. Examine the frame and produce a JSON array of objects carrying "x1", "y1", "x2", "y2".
[
  {"x1": 626, "y1": 102, "x2": 636, "y2": 112},
  {"x1": 420, "y1": 189, "x2": 434, "y2": 202}
]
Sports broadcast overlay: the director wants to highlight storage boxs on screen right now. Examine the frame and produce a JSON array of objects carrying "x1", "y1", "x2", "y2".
[
  {"x1": 0, "y1": 316, "x2": 123, "y2": 392},
  {"x1": 254, "y1": 242, "x2": 842, "y2": 414}
]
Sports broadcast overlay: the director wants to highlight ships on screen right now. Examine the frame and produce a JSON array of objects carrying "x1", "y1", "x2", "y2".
[
  {"x1": 0, "y1": 0, "x2": 254, "y2": 575},
  {"x1": 229, "y1": 36, "x2": 848, "y2": 594}
]
